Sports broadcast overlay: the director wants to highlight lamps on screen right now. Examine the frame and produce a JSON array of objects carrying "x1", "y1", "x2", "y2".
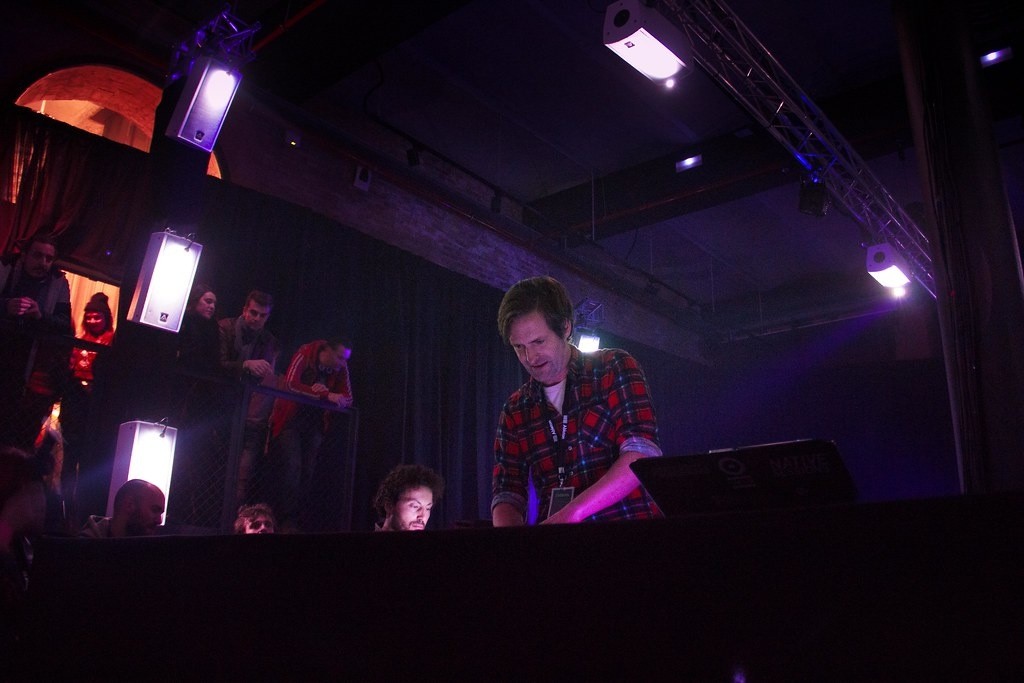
[
  {"x1": 578, "y1": 334, "x2": 600, "y2": 354},
  {"x1": 796, "y1": 170, "x2": 833, "y2": 215}
]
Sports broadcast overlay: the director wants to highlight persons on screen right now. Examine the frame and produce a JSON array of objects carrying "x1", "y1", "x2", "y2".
[
  {"x1": 272, "y1": 335, "x2": 353, "y2": 533},
  {"x1": 71, "y1": 293, "x2": 113, "y2": 388},
  {"x1": 162, "y1": 284, "x2": 277, "y2": 525},
  {"x1": 74, "y1": 479, "x2": 165, "y2": 537},
  {"x1": 375, "y1": 464, "x2": 439, "y2": 532},
  {"x1": 0, "y1": 229, "x2": 72, "y2": 446},
  {"x1": 235, "y1": 503, "x2": 275, "y2": 534},
  {"x1": 490, "y1": 276, "x2": 662, "y2": 527}
]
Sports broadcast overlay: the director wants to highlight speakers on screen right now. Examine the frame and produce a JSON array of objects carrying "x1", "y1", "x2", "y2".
[
  {"x1": 106, "y1": 421, "x2": 178, "y2": 526},
  {"x1": 165, "y1": 56, "x2": 244, "y2": 152},
  {"x1": 603, "y1": 0, "x2": 695, "y2": 86},
  {"x1": 127, "y1": 230, "x2": 204, "y2": 334},
  {"x1": 866, "y1": 243, "x2": 911, "y2": 288}
]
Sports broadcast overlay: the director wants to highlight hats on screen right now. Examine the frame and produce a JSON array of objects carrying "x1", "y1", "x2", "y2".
[{"x1": 85, "y1": 292, "x2": 109, "y2": 313}]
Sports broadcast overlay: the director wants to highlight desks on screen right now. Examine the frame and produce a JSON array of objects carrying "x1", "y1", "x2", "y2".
[{"x1": 22, "y1": 494, "x2": 1024, "y2": 683}]
